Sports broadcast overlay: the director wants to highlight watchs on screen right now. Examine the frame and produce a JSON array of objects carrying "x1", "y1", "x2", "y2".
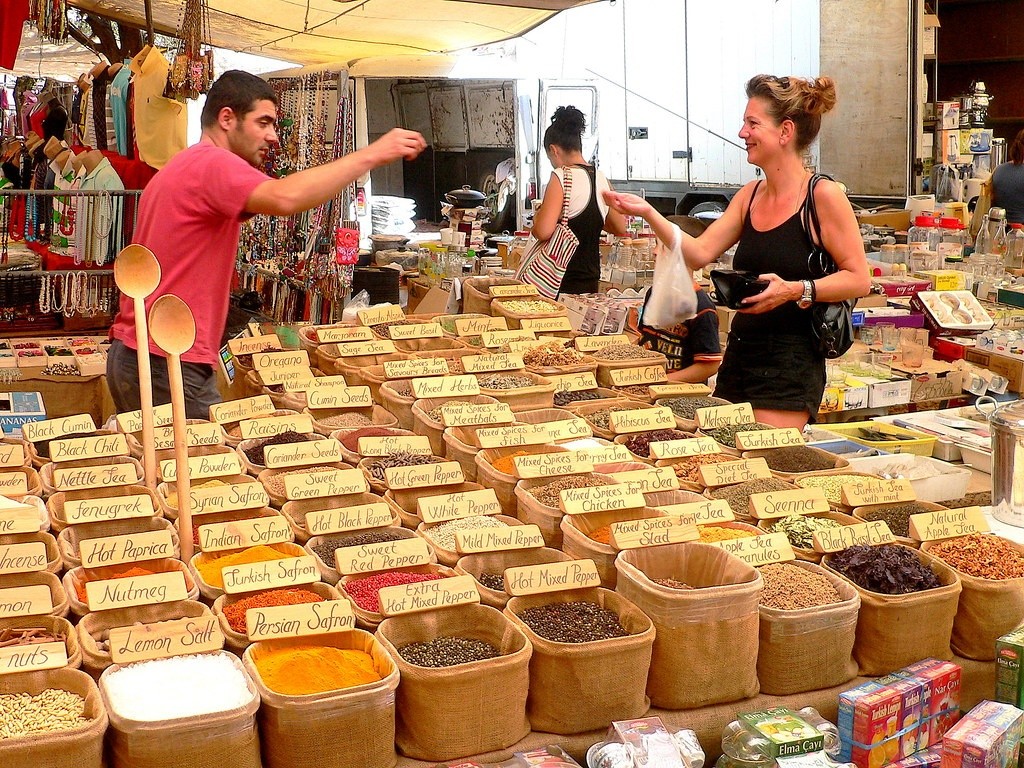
[{"x1": 795, "y1": 278, "x2": 812, "y2": 309}]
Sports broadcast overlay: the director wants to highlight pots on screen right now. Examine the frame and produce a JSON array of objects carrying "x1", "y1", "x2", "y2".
[{"x1": 444, "y1": 185, "x2": 487, "y2": 208}]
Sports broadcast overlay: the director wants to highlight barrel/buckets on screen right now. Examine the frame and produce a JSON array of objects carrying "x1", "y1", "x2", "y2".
[{"x1": 976, "y1": 396, "x2": 1024, "y2": 528}]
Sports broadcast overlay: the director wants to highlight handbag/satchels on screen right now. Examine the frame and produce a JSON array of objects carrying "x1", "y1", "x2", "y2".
[
  {"x1": 514, "y1": 165, "x2": 579, "y2": 299},
  {"x1": 805, "y1": 172, "x2": 858, "y2": 359},
  {"x1": 642, "y1": 223, "x2": 698, "y2": 331},
  {"x1": 969, "y1": 165, "x2": 998, "y2": 237}
]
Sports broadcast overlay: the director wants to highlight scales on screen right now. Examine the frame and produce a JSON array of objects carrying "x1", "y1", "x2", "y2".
[{"x1": 217, "y1": 321, "x2": 314, "y2": 389}]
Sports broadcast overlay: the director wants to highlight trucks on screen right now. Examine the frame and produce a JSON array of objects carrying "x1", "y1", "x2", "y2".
[{"x1": 514, "y1": 0, "x2": 942, "y2": 228}]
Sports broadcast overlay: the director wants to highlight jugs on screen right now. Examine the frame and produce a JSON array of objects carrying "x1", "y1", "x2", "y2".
[
  {"x1": 968, "y1": 133, "x2": 980, "y2": 149},
  {"x1": 980, "y1": 131, "x2": 990, "y2": 148},
  {"x1": 947, "y1": 135, "x2": 958, "y2": 162}
]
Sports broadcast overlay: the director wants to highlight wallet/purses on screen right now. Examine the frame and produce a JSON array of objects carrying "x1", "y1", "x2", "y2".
[{"x1": 710, "y1": 268, "x2": 770, "y2": 309}]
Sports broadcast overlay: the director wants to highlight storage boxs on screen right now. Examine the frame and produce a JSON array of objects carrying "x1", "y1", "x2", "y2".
[
  {"x1": 923, "y1": 14, "x2": 942, "y2": 59},
  {"x1": 0, "y1": 101, "x2": 1024, "y2": 768}
]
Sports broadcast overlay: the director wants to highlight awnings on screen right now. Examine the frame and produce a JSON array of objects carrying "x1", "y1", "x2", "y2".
[{"x1": 37, "y1": 0, "x2": 614, "y2": 66}]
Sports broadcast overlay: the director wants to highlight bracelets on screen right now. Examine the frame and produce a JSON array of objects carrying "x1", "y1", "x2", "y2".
[{"x1": 808, "y1": 278, "x2": 816, "y2": 305}]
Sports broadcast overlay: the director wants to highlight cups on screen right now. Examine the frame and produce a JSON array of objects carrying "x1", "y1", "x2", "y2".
[
  {"x1": 644, "y1": 279, "x2": 653, "y2": 298},
  {"x1": 440, "y1": 227, "x2": 453, "y2": 244},
  {"x1": 452, "y1": 232, "x2": 466, "y2": 247},
  {"x1": 825, "y1": 322, "x2": 929, "y2": 386},
  {"x1": 969, "y1": 253, "x2": 1011, "y2": 287}
]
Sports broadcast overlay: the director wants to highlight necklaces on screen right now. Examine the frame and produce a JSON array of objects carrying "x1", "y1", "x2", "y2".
[{"x1": 0, "y1": 271, "x2": 120, "y2": 323}]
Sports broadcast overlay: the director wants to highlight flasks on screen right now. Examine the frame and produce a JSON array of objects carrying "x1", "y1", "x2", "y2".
[
  {"x1": 988, "y1": 207, "x2": 1006, "y2": 236},
  {"x1": 991, "y1": 137, "x2": 1005, "y2": 172}
]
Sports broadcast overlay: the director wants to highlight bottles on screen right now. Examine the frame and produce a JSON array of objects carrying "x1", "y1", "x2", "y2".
[
  {"x1": 992, "y1": 221, "x2": 1006, "y2": 258},
  {"x1": 1004, "y1": 223, "x2": 1024, "y2": 268},
  {"x1": 859, "y1": 224, "x2": 973, "y2": 271},
  {"x1": 418, "y1": 244, "x2": 482, "y2": 288},
  {"x1": 608, "y1": 225, "x2": 657, "y2": 271},
  {"x1": 975, "y1": 214, "x2": 990, "y2": 254}
]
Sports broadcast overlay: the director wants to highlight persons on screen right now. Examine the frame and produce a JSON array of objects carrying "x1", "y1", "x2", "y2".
[
  {"x1": 636, "y1": 216, "x2": 723, "y2": 386},
  {"x1": 0, "y1": 43, "x2": 187, "y2": 263},
  {"x1": 530, "y1": 104, "x2": 626, "y2": 301},
  {"x1": 107, "y1": 70, "x2": 428, "y2": 421},
  {"x1": 601, "y1": 75, "x2": 870, "y2": 435}
]
